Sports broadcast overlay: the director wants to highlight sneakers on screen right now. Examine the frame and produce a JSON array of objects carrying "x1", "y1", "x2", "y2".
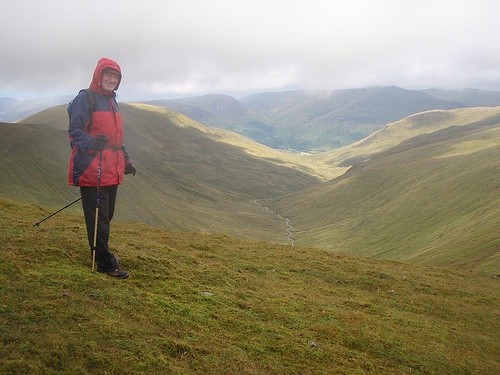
[{"x1": 106, "y1": 267, "x2": 129, "y2": 279}]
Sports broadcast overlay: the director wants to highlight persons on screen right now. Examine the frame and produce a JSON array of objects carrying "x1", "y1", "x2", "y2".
[{"x1": 67, "y1": 57, "x2": 137, "y2": 280}]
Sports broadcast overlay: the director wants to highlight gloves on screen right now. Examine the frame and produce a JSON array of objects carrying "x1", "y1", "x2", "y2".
[
  {"x1": 94, "y1": 135, "x2": 107, "y2": 150},
  {"x1": 124, "y1": 164, "x2": 136, "y2": 176}
]
